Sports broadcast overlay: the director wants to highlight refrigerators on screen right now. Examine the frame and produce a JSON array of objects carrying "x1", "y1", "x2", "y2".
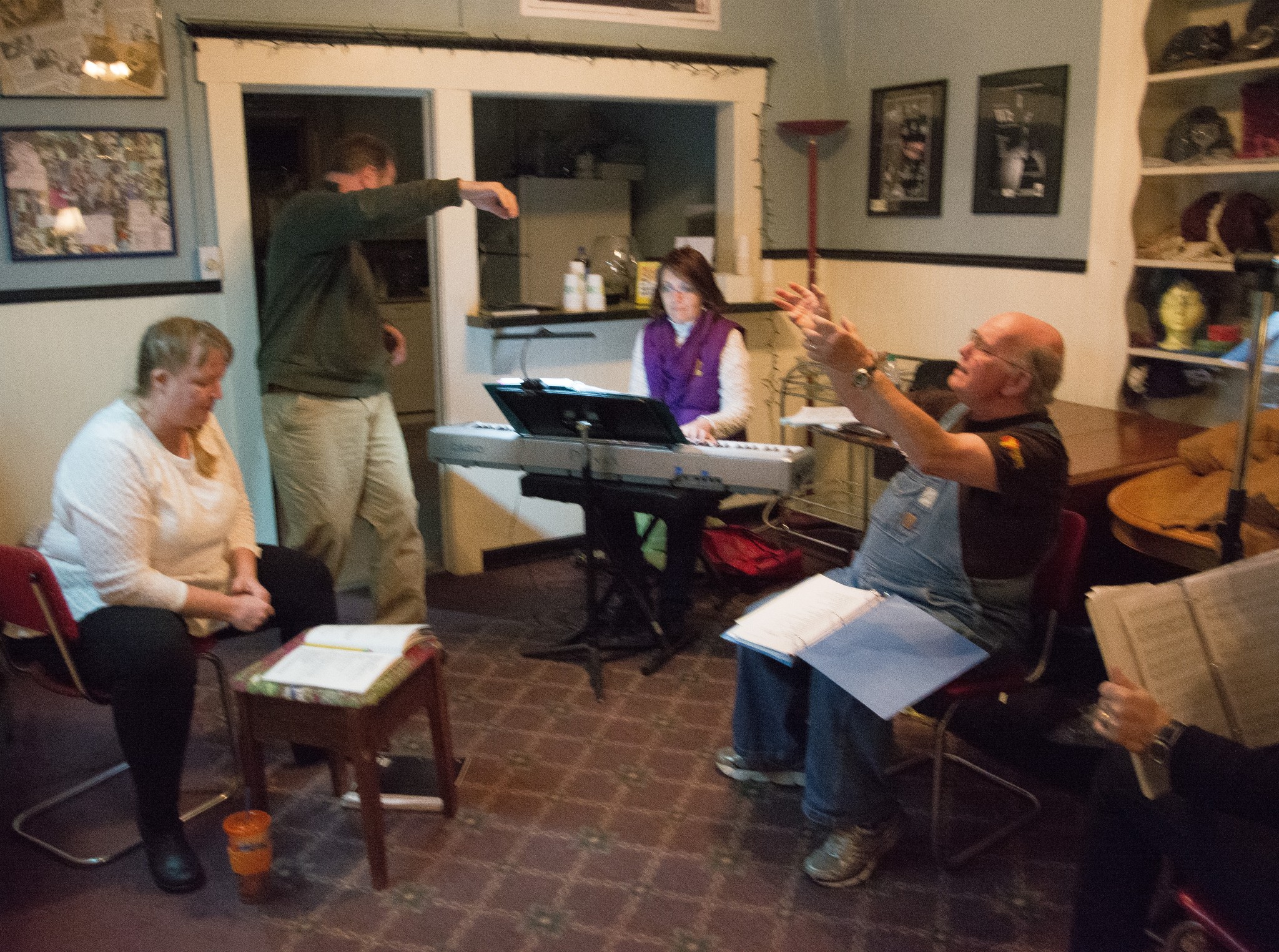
[{"x1": 478, "y1": 170, "x2": 631, "y2": 307}]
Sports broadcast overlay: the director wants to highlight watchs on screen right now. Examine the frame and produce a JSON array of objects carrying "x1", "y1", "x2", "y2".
[
  {"x1": 1144, "y1": 718, "x2": 1189, "y2": 769},
  {"x1": 847, "y1": 360, "x2": 881, "y2": 390}
]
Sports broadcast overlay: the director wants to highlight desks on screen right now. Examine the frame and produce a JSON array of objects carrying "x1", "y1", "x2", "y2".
[
  {"x1": 814, "y1": 386, "x2": 1210, "y2": 492},
  {"x1": 1107, "y1": 466, "x2": 1279, "y2": 574}
]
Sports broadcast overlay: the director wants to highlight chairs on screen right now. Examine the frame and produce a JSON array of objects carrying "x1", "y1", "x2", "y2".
[
  {"x1": 879, "y1": 505, "x2": 1087, "y2": 869},
  {"x1": 1, "y1": 541, "x2": 246, "y2": 869}
]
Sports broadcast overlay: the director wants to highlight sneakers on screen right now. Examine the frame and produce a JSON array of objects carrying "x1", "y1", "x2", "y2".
[
  {"x1": 715, "y1": 744, "x2": 807, "y2": 788},
  {"x1": 805, "y1": 802, "x2": 909, "y2": 888}
]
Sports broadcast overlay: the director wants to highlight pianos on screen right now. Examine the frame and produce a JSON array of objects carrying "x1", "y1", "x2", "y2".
[{"x1": 430, "y1": 421, "x2": 814, "y2": 496}]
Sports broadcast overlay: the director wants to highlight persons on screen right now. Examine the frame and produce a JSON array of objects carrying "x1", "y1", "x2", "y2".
[
  {"x1": 566, "y1": 247, "x2": 755, "y2": 572},
  {"x1": 30, "y1": 315, "x2": 365, "y2": 898},
  {"x1": 242, "y1": 133, "x2": 522, "y2": 639},
  {"x1": 1084, "y1": 622, "x2": 1277, "y2": 949},
  {"x1": 704, "y1": 281, "x2": 1071, "y2": 888}
]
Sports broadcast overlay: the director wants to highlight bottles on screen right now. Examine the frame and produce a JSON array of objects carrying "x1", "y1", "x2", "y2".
[{"x1": 575, "y1": 246, "x2": 590, "y2": 276}]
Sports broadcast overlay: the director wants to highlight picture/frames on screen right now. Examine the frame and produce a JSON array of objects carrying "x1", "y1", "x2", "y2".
[
  {"x1": 1, "y1": 0, "x2": 170, "y2": 101},
  {"x1": 968, "y1": 63, "x2": 1071, "y2": 216},
  {"x1": 865, "y1": 77, "x2": 949, "y2": 218},
  {"x1": 1, "y1": 122, "x2": 181, "y2": 262}
]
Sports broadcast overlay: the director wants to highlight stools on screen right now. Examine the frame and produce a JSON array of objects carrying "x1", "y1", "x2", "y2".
[{"x1": 232, "y1": 627, "x2": 459, "y2": 891}]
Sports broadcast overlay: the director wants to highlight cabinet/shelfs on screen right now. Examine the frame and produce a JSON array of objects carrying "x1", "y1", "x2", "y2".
[{"x1": 1111, "y1": 1, "x2": 1279, "y2": 427}]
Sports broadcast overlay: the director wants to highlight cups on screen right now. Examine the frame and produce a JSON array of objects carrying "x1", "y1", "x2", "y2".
[
  {"x1": 224, "y1": 809, "x2": 279, "y2": 905},
  {"x1": 561, "y1": 259, "x2": 607, "y2": 315}
]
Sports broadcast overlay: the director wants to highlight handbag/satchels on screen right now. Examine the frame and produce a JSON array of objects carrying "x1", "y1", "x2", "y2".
[{"x1": 696, "y1": 524, "x2": 805, "y2": 582}]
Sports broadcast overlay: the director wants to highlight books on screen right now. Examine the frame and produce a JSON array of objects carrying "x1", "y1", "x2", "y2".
[
  {"x1": 1083, "y1": 544, "x2": 1279, "y2": 800},
  {"x1": 259, "y1": 620, "x2": 438, "y2": 695},
  {"x1": 718, "y1": 573, "x2": 990, "y2": 722}
]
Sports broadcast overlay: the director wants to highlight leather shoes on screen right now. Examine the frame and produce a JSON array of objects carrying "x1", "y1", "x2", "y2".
[
  {"x1": 137, "y1": 817, "x2": 206, "y2": 892},
  {"x1": 292, "y1": 740, "x2": 328, "y2": 765}
]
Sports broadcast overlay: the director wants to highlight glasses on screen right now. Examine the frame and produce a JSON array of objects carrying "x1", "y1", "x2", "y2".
[
  {"x1": 658, "y1": 284, "x2": 698, "y2": 294},
  {"x1": 969, "y1": 332, "x2": 1033, "y2": 379}
]
